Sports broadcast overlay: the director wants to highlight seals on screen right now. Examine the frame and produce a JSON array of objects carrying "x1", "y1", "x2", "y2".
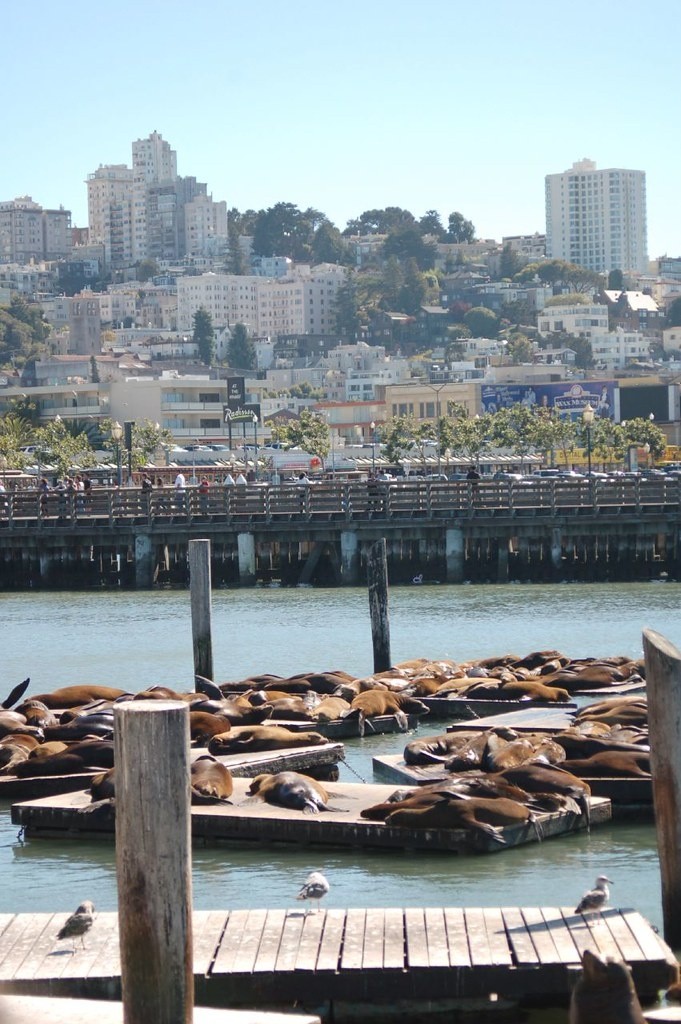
[
  {"x1": 238, "y1": 770, "x2": 357, "y2": 815},
  {"x1": 0, "y1": 651, "x2": 645, "y2": 809},
  {"x1": 358, "y1": 695, "x2": 651, "y2": 846}
]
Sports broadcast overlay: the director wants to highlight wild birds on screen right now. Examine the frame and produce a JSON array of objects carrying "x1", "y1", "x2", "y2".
[
  {"x1": 56, "y1": 901, "x2": 95, "y2": 954},
  {"x1": 296, "y1": 870, "x2": 329, "y2": 915},
  {"x1": 575, "y1": 875, "x2": 615, "y2": 929}
]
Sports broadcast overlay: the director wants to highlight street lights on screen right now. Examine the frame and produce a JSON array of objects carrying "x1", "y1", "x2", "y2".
[
  {"x1": 253, "y1": 415, "x2": 258, "y2": 484},
  {"x1": 371, "y1": 421, "x2": 375, "y2": 479},
  {"x1": 111, "y1": 421, "x2": 123, "y2": 487},
  {"x1": 583, "y1": 405, "x2": 594, "y2": 480},
  {"x1": 35, "y1": 444, "x2": 43, "y2": 484}
]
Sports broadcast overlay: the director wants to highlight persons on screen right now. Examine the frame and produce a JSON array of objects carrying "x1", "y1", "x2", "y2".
[
  {"x1": 295, "y1": 472, "x2": 311, "y2": 513},
  {"x1": 174, "y1": 469, "x2": 185, "y2": 515},
  {"x1": 0, "y1": 480, "x2": 18, "y2": 520},
  {"x1": 140, "y1": 473, "x2": 152, "y2": 517},
  {"x1": 37, "y1": 472, "x2": 91, "y2": 519},
  {"x1": 466, "y1": 464, "x2": 481, "y2": 502},
  {"x1": 197, "y1": 477, "x2": 209, "y2": 515},
  {"x1": 154, "y1": 478, "x2": 167, "y2": 516},
  {"x1": 364, "y1": 470, "x2": 389, "y2": 514}
]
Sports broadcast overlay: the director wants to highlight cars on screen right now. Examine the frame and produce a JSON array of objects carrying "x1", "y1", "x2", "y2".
[{"x1": 0, "y1": 442, "x2": 681, "y2": 486}]
